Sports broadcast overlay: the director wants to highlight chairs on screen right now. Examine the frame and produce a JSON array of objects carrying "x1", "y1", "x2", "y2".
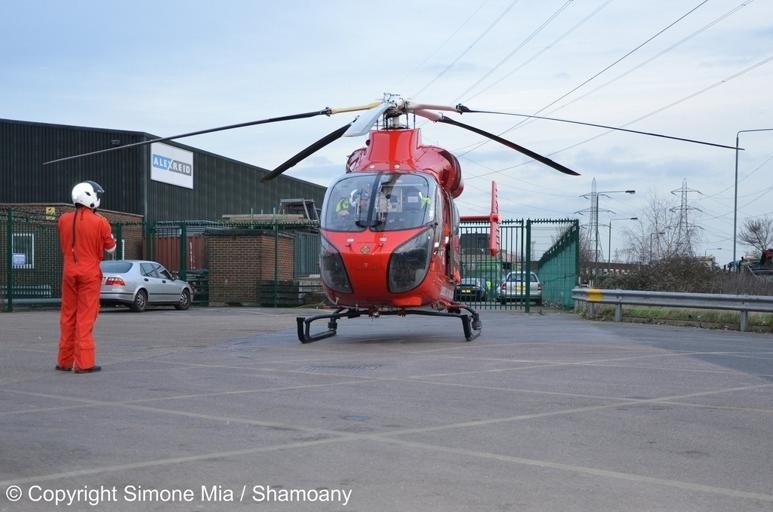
[{"x1": 404, "y1": 186, "x2": 424, "y2": 225}]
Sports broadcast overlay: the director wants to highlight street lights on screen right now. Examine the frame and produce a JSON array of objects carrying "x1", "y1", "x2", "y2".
[{"x1": 594, "y1": 189, "x2": 638, "y2": 260}]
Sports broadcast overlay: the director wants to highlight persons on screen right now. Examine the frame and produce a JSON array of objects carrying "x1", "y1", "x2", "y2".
[
  {"x1": 53, "y1": 179, "x2": 115, "y2": 373},
  {"x1": 337, "y1": 189, "x2": 378, "y2": 233}
]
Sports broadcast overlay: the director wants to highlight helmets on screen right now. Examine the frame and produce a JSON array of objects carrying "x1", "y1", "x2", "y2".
[
  {"x1": 71, "y1": 181, "x2": 105, "y2": 209},
  {"x1": 349, "y1": 189, "x2": 369, "y2": 209}
]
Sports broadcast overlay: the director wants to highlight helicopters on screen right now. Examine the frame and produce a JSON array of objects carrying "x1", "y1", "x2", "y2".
[{"x1": 43, "y1": 86, "x2": 746, "y2": 342}]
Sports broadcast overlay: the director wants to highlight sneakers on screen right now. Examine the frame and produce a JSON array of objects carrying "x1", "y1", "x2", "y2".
[{"x1": 56, "y1": 365, "x2": 101, "y2": 373}]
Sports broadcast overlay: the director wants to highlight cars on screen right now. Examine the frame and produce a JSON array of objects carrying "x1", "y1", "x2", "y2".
[
  {"x1": 453, "y1": 278, "x2": 487, "y2": 303},
  {"x1": 95, "y1": 260, "x2": 194, "y2": 311},
  {"x1": 496, "y1": 270, "x2": 543, "y2": 306}
]
